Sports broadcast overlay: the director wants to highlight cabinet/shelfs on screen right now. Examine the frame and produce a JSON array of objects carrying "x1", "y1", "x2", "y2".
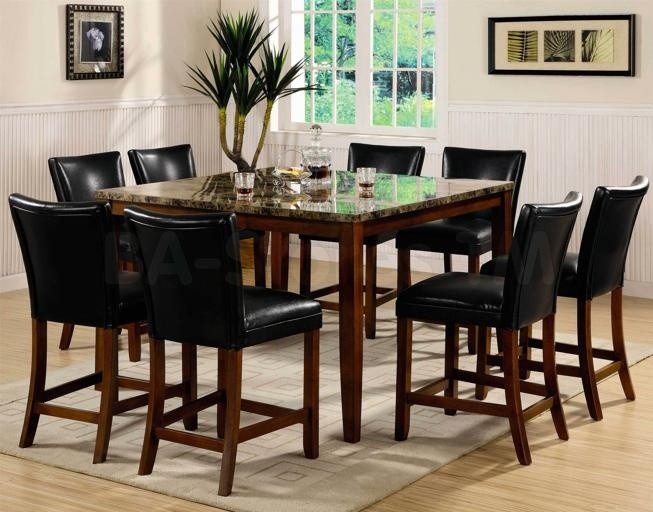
[
  {"x1": 301, "y1": 185, "x2": 331, "y2": 212},
  {"x1": 302, "y1": 126, "x2": 333, "y2": 190}
]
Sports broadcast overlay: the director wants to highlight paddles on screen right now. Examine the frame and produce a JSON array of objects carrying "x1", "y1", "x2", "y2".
[{"x1": 0, "y1": 313, "x2": 653, "y2": 511}]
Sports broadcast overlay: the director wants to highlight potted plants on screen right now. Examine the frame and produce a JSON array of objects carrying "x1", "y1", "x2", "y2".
[
  {"x1": 65, "y1": 2, "x2": 126, "y2": 80},
  {"x1": 486, "y1": 14, "x2": 636, "y2": 78}
]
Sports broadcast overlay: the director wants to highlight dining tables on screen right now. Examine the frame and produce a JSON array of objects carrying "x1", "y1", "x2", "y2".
[{"x1": 181, "y1": 1, "x2": 329, "y2": 273}]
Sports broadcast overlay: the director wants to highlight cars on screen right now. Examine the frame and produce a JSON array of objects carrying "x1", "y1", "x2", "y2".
[
  {"x1": 233, "y1": 171, "x2": 255, "y2": 199},
  {"x1": 355, "y1": 167, "x2": 376, "y2": 193},
  {"x1": 356, "y1": 193, "x2": 375, "y2": 214},
  {"x1": 235, "y1": 199, "x2": 252, "y2": 206}
]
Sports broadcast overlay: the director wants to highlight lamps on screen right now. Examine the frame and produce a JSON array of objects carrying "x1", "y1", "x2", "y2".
[
  {"x1": 6, "y1": 192, "x2": 199, "y2": 464},
  {"x1": 473, "y1": 173, "x2": 649, "y2": 421},
  {"x1": 397, "y1": 147, "x2": 529, "y2": 355},
  {"x1": 120, "y1": 202, "x2": 324, "y2": 498},
  {"x1": 128, "y1": 144, "x2": 199, "y2": 184},
  {"x1": 393, "y1": 190, "x2": 586, "y2": 469},
  {"x1": 47, "y1": 142, "x2": 152, "y2": 365},
  {"x1": 296, "y1": 143, "x2": 428, "y2": 340}
]
